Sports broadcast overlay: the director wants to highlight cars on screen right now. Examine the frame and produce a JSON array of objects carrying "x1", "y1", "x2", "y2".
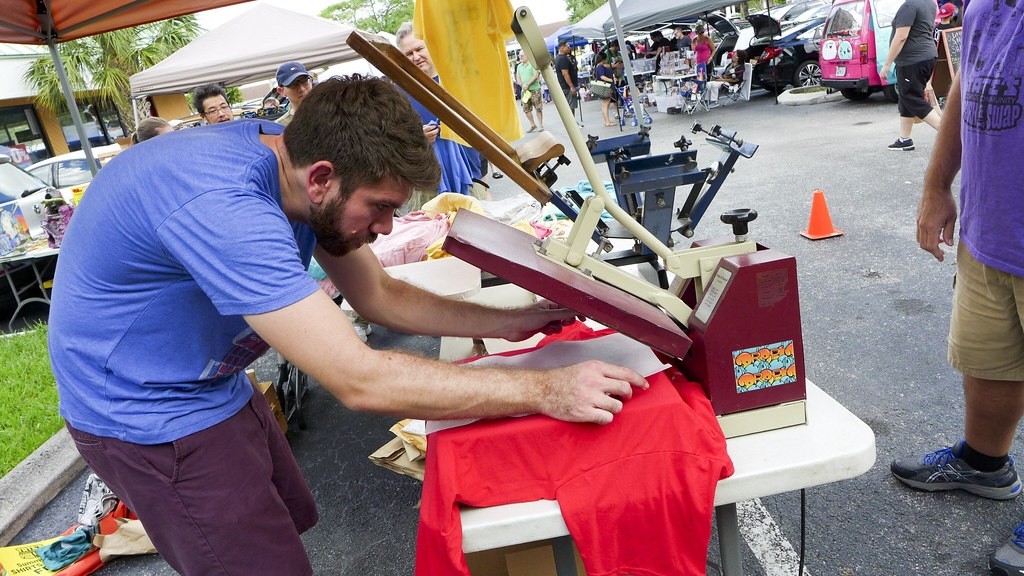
[
  {"x1": 0, "y1": 109, "x2": 256, "y2": 315},
  {"x1": 624, "y1": 0, "x2": 942, "y2": 103}
]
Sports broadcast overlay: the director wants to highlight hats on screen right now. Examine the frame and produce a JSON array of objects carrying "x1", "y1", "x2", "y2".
[
  {"x1": 936, "y1": 3, "x2": 955, "y2": 18},
  {"x1": 276, "y1": 62, "x2": 313, "y2": 86}
]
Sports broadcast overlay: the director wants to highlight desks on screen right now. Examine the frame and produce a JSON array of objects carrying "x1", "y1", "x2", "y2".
[
  {"x1": 654, "y1": 74, "x2": 697, "y2": 96},
  {"x1": 424, "y1": 281, "x2": 877, "y2": 576},
  {"x1": 0, "y1": 238, "x2": 59, "y2": 333}
]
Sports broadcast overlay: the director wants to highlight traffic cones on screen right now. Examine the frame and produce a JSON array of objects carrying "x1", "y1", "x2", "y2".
[{"x1": 799, "y1": 188, "x2": 844, "y2": 240}]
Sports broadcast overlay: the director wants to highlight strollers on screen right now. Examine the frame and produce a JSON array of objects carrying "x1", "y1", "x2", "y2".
[{"x1": 613, "y1": 74, "x2": 652, "y2": 127}]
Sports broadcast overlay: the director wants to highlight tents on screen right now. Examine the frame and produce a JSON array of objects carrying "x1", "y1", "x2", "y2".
[
  {"x1": 571, "y1": 0, "x2": 779, "y2": 132},
  {"x1": 129, "y1": 0, "x2": 391, "y2": 128},
  {"x1": 544, "y1": 26, "x2": 590, "y2": 64}
]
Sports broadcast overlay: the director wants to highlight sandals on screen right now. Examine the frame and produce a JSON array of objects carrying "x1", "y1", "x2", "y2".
[{"x1": 605, "y1": 122, "x2": 617, "y2": 126}]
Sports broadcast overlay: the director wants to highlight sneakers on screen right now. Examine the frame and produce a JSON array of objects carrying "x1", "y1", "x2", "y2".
[
  {"x1": 891, "y1": 440, "x2": 1022, "y2": 500},
  {"x1": 988, "y1": 522, "x2": 1024, "y2": 576},
  {"x1": 888, "y1": 137, "x2": 915, "y2": 151}
]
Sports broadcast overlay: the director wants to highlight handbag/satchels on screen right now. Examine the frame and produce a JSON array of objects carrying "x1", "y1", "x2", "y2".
[
  {"x1": 589, "y1": 66, "x2": 611, "y2": 99},
  {"x1": 913, "y1": 82, "x2": 941, "y2": 124}
]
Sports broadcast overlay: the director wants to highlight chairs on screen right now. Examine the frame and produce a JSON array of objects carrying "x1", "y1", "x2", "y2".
[{"x1": 677, "y1": 62, "x2": 753, "y2": 115}]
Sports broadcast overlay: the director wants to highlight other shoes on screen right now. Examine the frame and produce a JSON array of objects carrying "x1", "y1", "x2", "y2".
[
  {"x1": 538, "y1": 126, "x2": 544, "y2": 132},
  {"x1": 493, "y1": 172, "x2": 503, "y2": 178},
  {"x1": 709, "y1": 102, "x2": 719, "y2": 108},
  {"x1": 527, "y1": 126, "x2": 537, "y2": 133}
]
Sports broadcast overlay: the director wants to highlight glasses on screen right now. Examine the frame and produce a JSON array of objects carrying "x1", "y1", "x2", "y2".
[
  {"x1": 698, "y1": 31, "x2": 704, "y2": 34},
  {"x1": 202, "y1": 105, "x2": 229, "y2": 117},
  {"x1": 284, "y1": 77, "x2": 312, "y2": 89}
]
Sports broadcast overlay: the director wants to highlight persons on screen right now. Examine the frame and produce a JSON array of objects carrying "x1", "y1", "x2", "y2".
[
  {"x1": 275, "y1": 62, "x2": 313, "y2": 127},
  {"x1": 192, "y1": 84, "x2": 233, "y2": 126},
  {"x1": 880, "y1": 0, "x2": 962, "y2": 151},
  {"x1": 509, "y1": 24, "x2": 747, "y2": 131},
  {"x1": 47, "y1": 73, "x2": 649, "y2": 576},
  {"x1": 890, "y1": 0, "x2": 1024, "y2": 576},
  {"x1": 131, "y1": 117, "x2": 175, "y2": 147},
  {"x1": 377, "y1": 21, "x2": 503, "y2": 215}
]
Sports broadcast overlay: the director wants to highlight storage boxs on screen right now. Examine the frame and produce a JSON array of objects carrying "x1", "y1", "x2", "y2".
[{"x1": 631, "y1": 58, "x2": 656, "y2": 73}]
